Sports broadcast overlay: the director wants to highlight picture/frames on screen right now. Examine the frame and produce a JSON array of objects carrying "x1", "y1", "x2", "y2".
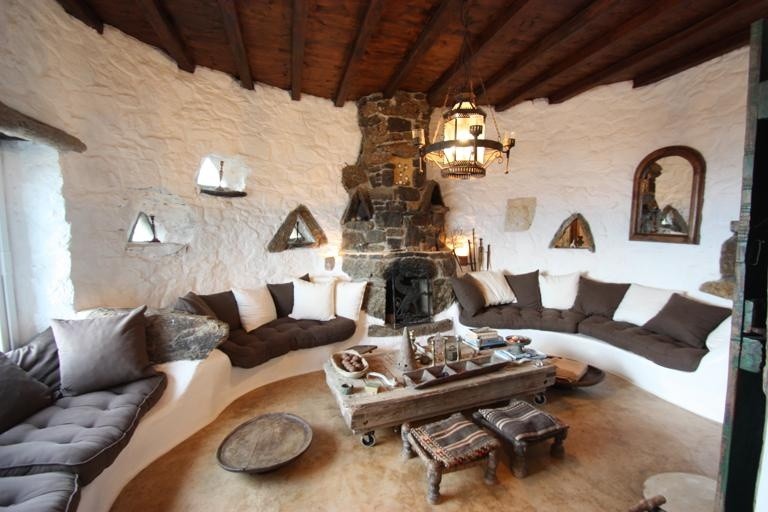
[{"x1": 628, "y1": 145, "x2": 705, "y2": 246}]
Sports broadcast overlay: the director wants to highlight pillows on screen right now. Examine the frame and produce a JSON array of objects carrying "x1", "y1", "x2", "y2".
[
  {"x1": 175, "y1": 291, "x2": 222, "y2": 320},
  {"x1": 49, "y1": 306, "x2": 155, "y2": 393},
  {"x1": 200, "y1": 290, "x2": 239, "y2": 335},
  {"x1": 539, "y1": 276, "x2": 584, "y2": 309},
  {"x1": 453, "y1": 274, "x2": 485, "y2": 318},
  {"x1": 644, "y1": 293, "x2": 731, "y2": 348},
  {"x1": 572, "y1": 276, "x2": 630, "y2": 320},
  {"x1": 469, "y1": 268, "x2": 517, "y2": 307},
  {"x1": 613, "y1": 284, "x2": 686, "y2": 330},
  {"x1": 288, "y1": 278, "x2": 337, "y2": 320},
  {"x1": 505, "y1": 269, "x2": 546, "y2": 309},
  {"x1": 268, "y1": 273, "x2": 319, "y2": 317},
  {"x1": 1, "y1": 351, "x2": 55, "y2": 433},
  {"x1": 314, "y1": 277, "x2": 368, "y2": 320},
  {"x1": 232, "y1": 280, "x2": 283, "y2": 332}
]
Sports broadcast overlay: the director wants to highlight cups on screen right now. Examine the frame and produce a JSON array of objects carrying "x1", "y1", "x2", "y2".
[{"x1": 340, "y1": 383, "x2": 353, "y2": 395}]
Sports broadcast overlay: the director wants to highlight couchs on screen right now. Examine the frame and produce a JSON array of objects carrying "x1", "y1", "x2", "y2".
[
  {"x1": 434, "y1": 270, "x2": 733, "y2": 426},
  {"x1": 0, "y1": 271, "x2": 385, "y2": 512}
]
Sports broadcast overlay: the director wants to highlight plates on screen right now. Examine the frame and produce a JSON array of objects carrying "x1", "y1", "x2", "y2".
[
  {"x1": 504, "y1": 335, "x2": 532, "y2": 354},
  {"x1": 329, "y1": 350, "x2": 370, "y2": 379}
]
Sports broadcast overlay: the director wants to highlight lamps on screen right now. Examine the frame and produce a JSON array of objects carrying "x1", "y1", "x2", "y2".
[{"x1": 420, "y1": 0, "x2": 515, "y2": 183}]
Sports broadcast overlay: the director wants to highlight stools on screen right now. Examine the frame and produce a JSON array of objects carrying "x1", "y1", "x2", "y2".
[
  {"x1": 404, "y1": 414, "x2": 498, "y2": 503},
  {"x1": 473, "y1": 399, "x2": 568, "y2": 479}
]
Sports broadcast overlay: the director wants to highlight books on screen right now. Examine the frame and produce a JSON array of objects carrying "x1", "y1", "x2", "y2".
[{"x1": 465, "y1": 326, "x2": 507, "y2": 350}]
